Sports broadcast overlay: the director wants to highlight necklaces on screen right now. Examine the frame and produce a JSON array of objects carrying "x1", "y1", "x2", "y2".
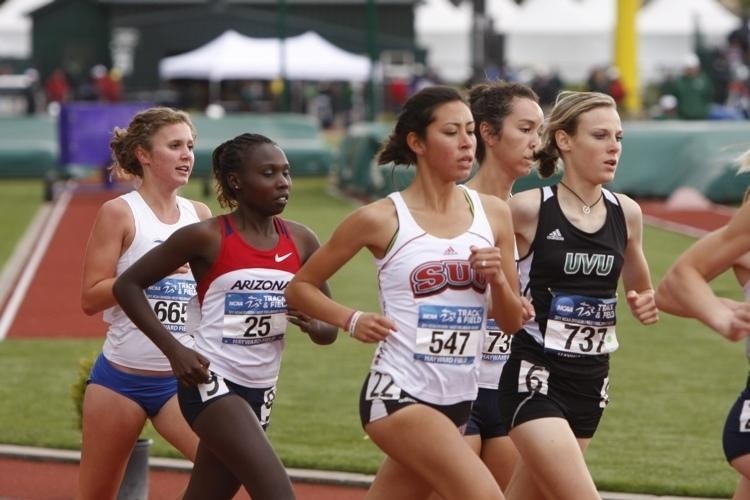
[{"x1": 559, "y1": 180, "x2": 602, "y2": 215}]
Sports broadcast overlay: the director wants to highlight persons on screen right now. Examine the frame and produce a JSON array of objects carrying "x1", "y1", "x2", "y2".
[
  {"x1": 484, "y1": 86, "x2": 662, "y2": 499},
  {"x1": 453, "y1": 78, "x2": 547, "y2": 500},
  {"x1": 665, "y1": 149, "x2": 749, "y2": 500},
  {"x1": 280, "y1": 82, "x2": 527, "y2": 499},
  {"x1": 73, "y1": 103, "x2": 214, "y2": 500},
  {"x1": 653, "y1": 186, "x2": 750, "y2": 326},
  {"x1": 112, "y1": 131, "x2": 340, "y2": 500},
  {"x1": 39, "y1": 30, "x2": 750, "y2": 125}
]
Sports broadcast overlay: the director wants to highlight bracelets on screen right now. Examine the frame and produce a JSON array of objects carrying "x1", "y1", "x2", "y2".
[
  {"x1": 348, "y1": 312, "x2": 363, "y2": 337},
  {"x1": 342, "y1": 307, "x2": 356, "y2": 330}
]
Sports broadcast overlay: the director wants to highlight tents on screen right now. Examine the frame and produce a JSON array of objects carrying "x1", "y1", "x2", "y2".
[
  {"x1": 157, "y1": 31, "x2": 285, "y2": 108},
  {"x1": 280, "y1": 32, "x2": 371, "y2": 81}
]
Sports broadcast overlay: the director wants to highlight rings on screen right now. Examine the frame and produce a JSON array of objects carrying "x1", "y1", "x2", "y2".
[{"x1": 481, "y1": 260, "x2": 487, "y2": 269}]
